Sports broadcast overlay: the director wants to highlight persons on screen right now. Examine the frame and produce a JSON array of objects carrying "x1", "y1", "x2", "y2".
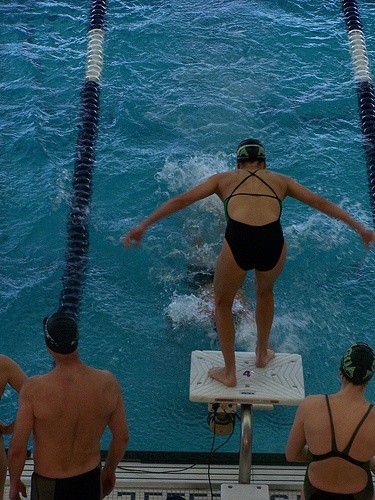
[
  {"x1": 0, "y1": 352, "x2": 29, "y2": 500},
  {"x1": 286, "y1": 339, "x2": 375, "y2": 500},
  {"x1": 118, "y1": 138, "x2": 375, "y2": 387},
  {"x1": 7, "y1": 312, "x2": 129, "y2": 500},
  {"x1": 170, "y1": 200, "x2": 247, "y2": 345}
]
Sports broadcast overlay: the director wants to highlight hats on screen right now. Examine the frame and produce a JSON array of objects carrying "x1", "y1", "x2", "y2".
[
  {"x1": 237, "y1": 139, "x2": 265, "y2": 160},
  {"x1": 44, "y1": 312, "x2": 78, "y2": 354},
  {"x1": 342, "y1": 342, "x2": 375, "y2": 384}
]
